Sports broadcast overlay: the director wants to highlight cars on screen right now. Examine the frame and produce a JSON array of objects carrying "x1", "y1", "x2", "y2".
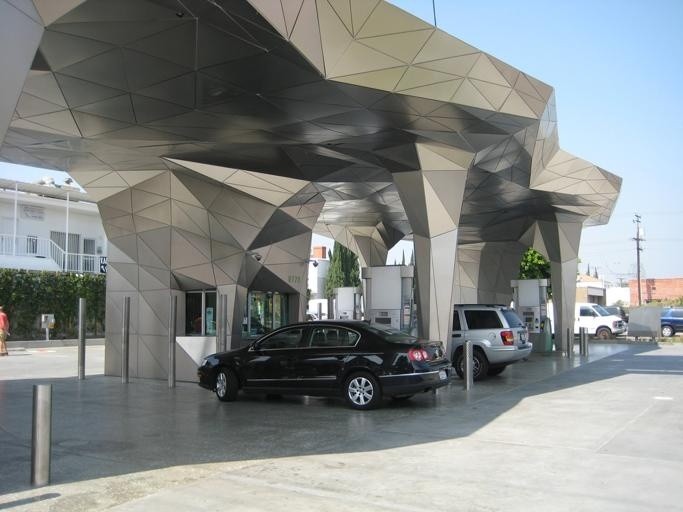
[
  {"x1": 198, "y1": 319, "x2": 452, "y2": 410},
  {"x1": 661, "y1": 307, "x2": 683, "y2": 336}
]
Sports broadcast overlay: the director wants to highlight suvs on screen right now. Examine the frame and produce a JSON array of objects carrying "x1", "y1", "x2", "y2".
[{"x1": 453, "y1": 302, "x2": 532, "y2": 380}]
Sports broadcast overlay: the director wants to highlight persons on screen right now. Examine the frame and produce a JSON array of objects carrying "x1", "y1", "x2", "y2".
[{"x1": 0, "y1": 306, "x2": 9, "y2": 356}]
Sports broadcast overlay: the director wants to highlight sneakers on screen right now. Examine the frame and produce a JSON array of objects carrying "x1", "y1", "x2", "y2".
[{"x1": 0, "y1": 351, "x2": 8, "y2": 356}]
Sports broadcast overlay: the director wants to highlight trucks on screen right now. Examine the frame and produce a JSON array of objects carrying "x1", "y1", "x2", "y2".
[{"x1": 511, "y1": 301, "x2": 627, "y2": 339}]
